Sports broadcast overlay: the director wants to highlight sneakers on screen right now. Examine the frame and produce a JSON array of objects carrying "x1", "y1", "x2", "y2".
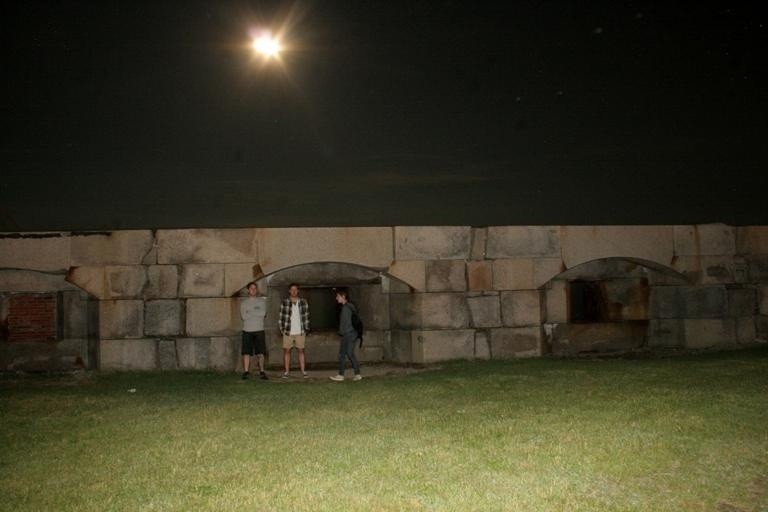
[
  {"x1": 330, "y1": 374, "x2": 346, "y2": 382},
  {"x1": 299, "y1": 372, "x2": 310, "y2": 380},
  {"x1": 352, "y1": 375, "x2": 363, "y2": 382},
  {"x1": 281, "y1": 371, "x2": 291, "y2": 380},
  {"x1": 242, "y1": 371, "x2": 252, "y2": 382},
  {"x1": 259, "y1": 371, "x2": 269, "y2": 381}
]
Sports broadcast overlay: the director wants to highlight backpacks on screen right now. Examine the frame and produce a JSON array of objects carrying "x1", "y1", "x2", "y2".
[{"x1": 345, "y1": 304, "x2": 363, "y2": 331}]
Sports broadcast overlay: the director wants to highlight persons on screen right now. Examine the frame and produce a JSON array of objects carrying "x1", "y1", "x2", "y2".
[
  {"x1": 276, "y1": 282, "x2": 313, "y2": 378},
  {"x1": 239, "y1": 282, "x2": 270, "y2": 381},
  {"x1": 328, "y1": 286, "x2": 363, "y2": 381}
]
